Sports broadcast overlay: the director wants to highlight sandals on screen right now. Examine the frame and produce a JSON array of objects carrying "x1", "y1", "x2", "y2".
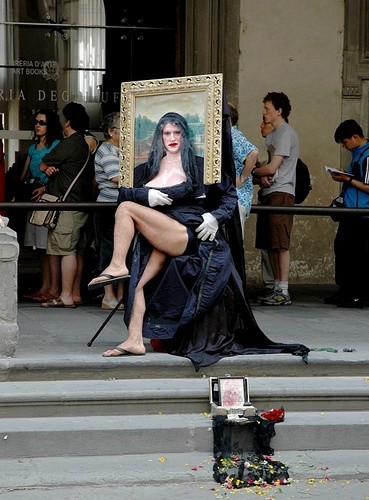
[
  {"x1": 33, "y1": 293, "x2": 57, "y2": 302},
  {"x1": 23, "y1": 291, "x2": 43, "y2": 300}
]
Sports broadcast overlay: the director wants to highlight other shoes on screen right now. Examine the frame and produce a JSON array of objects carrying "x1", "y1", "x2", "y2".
[
  {"x1": 336, "y1": 292, "x2": 369, "y2": 308},
  {"x1": 324, "y1": 291, "x2": 352, "y2": 304}
]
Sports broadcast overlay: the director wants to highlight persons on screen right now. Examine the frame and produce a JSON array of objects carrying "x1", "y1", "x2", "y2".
[
  {"x1": 14, "y1": 109, "x2": 63, "y2": 302},
  {"x1": 87, "y1": 172, "x2": 238, "y2": 357},
  {"x1": 254, "y1": 92, "x2": 300, "y2": 305},
  {"x1": 92, "y1": 112, "x2": 126, "y2": 310},
  {"x1": 252, "y1": 115, "x2": 289, "y2": 302},
  {"x1": 79, "y1": 115, "x2": 100, "y2": 204},
  {"x1": 39, "y1": 102, "x2": 91, "y2": 309},
  {"x1": 225, "y1": 102, "x2": 259, "y2": 248},
  {"x1": 334, "y1": 119, "x2": 369, "y2": 309}
]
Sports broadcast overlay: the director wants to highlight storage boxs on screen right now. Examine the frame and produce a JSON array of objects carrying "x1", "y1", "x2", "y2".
[{"x1": 209, "y1": 375, "x2": 257, "y2": 417}]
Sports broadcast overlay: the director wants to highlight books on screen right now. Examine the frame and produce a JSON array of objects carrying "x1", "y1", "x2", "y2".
[{"x1": 325, "y1": 165, "x2": 356, "y2": 179}]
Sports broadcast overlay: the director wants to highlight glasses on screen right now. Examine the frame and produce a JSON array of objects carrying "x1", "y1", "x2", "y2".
[{"x1": 30, "y1": 119, "x2": 47, "y2": 126}]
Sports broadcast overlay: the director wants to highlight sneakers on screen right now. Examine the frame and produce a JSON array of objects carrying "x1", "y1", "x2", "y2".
[
  {"x1": 259, "y1": 289, "x2": 292, "y2": 306},
  {"x1": 257, "y1": 287, "x2": 277, "y2": 302}
]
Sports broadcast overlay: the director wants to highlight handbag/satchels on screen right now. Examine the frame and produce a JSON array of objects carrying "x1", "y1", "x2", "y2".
[
  {"x1": 330, "y1": 196, "x2": 345, "y2": 223},
  {"x1": 13, "y1": 177, "x2": 44, "y2": 202},
  {"x1": 29, "y1": 194, "x2": 62, "y2": 229},
  {"x1": 294, "y1": 158, "x2": 312, "y2": 204}
]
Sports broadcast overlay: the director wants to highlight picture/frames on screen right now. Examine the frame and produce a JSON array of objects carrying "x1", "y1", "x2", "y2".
[{"x1": 118, "y1": 72, "x2": 222, "y2": 198}]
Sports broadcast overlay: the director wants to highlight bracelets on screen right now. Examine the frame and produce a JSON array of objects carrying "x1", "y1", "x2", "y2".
[{"x1": 346, "y1": 177, "x2": 353, "y2": 184}]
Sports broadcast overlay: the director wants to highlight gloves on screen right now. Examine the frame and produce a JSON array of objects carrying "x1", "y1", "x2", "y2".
[
  {"x1": 148, "y1": 189, "x2": 173, "y2": 207},
  {"x1": 195, "y1": 212, "x2": 218, "y2": 241}
]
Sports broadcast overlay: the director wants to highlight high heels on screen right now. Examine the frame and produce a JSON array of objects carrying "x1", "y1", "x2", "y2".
[{"x1": 102, "y1": 300, "x2": 124, "y2": 310}]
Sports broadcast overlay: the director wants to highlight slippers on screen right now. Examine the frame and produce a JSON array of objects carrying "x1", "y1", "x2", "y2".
[
  {"x1": 102, "y1": 346, "x2": 143, "y2": 357},
  {"x1": 74, "y1": 301, "x2": 83, "y2": 305},
  {"x1": 87, "y1": 274, "x2": 131, "y2": 289},
  {"x1": 40, "y1": 297, "x2": 76, "y2": 308}
]
